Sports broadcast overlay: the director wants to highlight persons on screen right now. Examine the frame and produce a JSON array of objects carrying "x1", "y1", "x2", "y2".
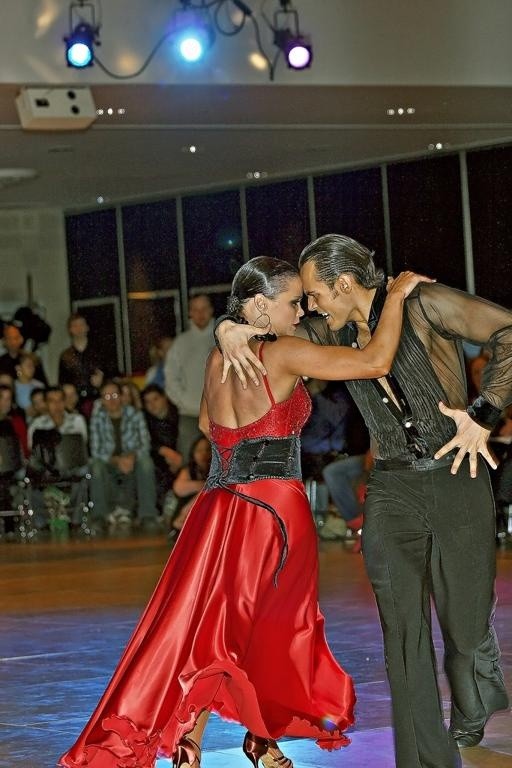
[
  {"x1": 28, "y1": 390, "x2": 88, "y2": 527},
  {"x1": 163, "y1": 291, "x2": 218, "y2": 483},
  {"x1": 2, "y1": 373, "x2": 23, "y2": 428},
  {"x1": 144, "y1": 337, "x2": 170, "y2": 387},
  {"x1": 10, "y1": 353, "x2": 43, "y2": 422},
  {"x1": 23, "y1": 388, "x2": 44, "y2": 422},
  {"x1": 59, "y1": 312, "x2": 108, "y2": 421},
  {"x1": 170, "y1": 436, "x2": 211, "y2": 539},
  {"x1": 142, "y1": 386, "x2": 182, "y2": 516},
  {"x1": 113, "y1": 379, "x2": 138, "y2": 408},
  {"x1": 55, "y1": 256, "x2": 439, "y2": 768},
  {"x1": 91, "y1": 383, "x2": 157, "y2": 532},
  {"x1": 1, "y1": 387, "x2": 19, "y2": 510},
  {"x1": 466, "y1": 354, "x2": 509, "y2": 540},
  {"x1": 214, "y1": 233, "x2": 512, "y2": 768},
  {"x1": 2, "y1": 326, "x2": 49, "y2": 384},
  {"x1": 323, "y1": 396, "x2": 383, "y2": 552},
  {"x1": 59, "y1": 383, "x2": 77, "y2": 413}
]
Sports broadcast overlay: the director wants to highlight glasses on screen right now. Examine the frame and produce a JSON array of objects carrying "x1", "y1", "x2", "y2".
[{"x1": 104, "y1": 392, "x2": 119, "y2": 401}]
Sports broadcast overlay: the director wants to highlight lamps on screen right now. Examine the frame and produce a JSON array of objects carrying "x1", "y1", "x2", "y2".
[
  {"x1": 61, "y1": 19, "x2": 101, "y2": 68},
  {"x1": 174, "y1": 0, "x2": 214, "y2": 62},
  {"x1": 258, "y1": 0, "x2": 313, "y2": 82}
]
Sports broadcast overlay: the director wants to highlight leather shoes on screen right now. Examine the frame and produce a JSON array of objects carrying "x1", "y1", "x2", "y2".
[{"x1": 448, "y1": 723, "x2": 484, "y2": 748}]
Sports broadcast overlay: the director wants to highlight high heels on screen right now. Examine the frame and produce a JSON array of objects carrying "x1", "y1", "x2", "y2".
[
  {"x1": 243, "y1": 732, "x2": 292, "y2": 768},
  {"x1": 174, "y1": 738, "x2": 200, "y2": 768}
]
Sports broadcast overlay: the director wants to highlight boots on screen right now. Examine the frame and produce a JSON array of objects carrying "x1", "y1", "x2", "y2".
[{"x1": 344, "y1": 515, "x2": 364, "y2": 554}]
